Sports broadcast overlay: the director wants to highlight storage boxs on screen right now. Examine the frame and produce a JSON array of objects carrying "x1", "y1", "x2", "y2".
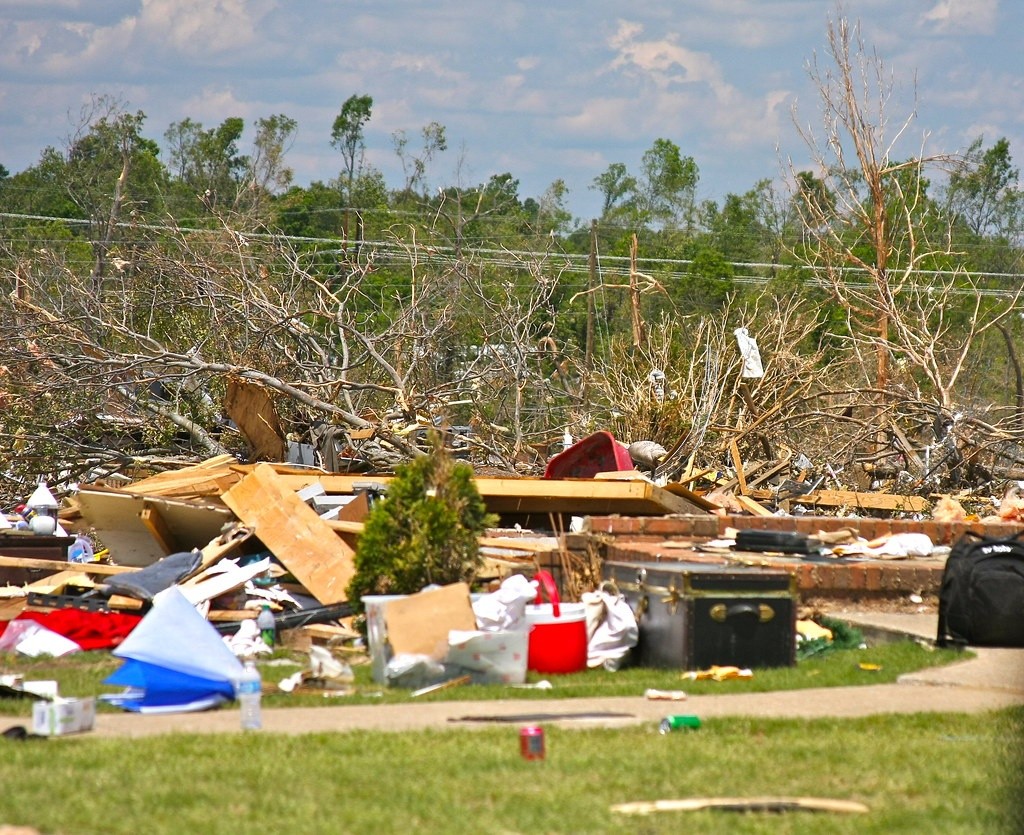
[
  {"x1": 543, "y1": 430, "x2": 634, "y2": 479},
  {"x1": 599, "y1": 560, "x2": 797, "y2": 672},
  {"x1": 360, "y1": 594, "x2": 400, "y2": 686}
]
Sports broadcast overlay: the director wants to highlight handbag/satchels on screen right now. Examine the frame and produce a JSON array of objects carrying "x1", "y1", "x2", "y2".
[{"x1": 936, "y1": 530, "x2": 1024, "y2": 647}]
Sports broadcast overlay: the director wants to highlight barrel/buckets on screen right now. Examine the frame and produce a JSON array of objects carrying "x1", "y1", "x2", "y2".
[{"x1": 526, "y1": 572, "x2": 587, "y2": 677}]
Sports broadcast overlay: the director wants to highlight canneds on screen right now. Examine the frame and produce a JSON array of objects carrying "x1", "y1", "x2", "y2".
[
  {"x1": 519, "y1": 726, "x2": 544, "y2": 760},
  {"x1": 659, "y1": 715, "x2": 701, "y2": 735}
]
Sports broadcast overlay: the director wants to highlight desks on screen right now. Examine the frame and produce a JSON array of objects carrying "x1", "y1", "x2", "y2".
[{"x1": 0, "y1": 535, "x2": 76, "y2": 585}]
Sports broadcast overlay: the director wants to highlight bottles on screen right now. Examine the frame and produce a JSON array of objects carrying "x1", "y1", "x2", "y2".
[
  {"x1": 26, "y1": 482, "x2": 58, "y2": 532},
  {"x1": 257, "y1": 606, "x2": 275, "y2": 648},
  {"x1": 239, "y1": 662, "x2": 262, "y2": 732}
]
